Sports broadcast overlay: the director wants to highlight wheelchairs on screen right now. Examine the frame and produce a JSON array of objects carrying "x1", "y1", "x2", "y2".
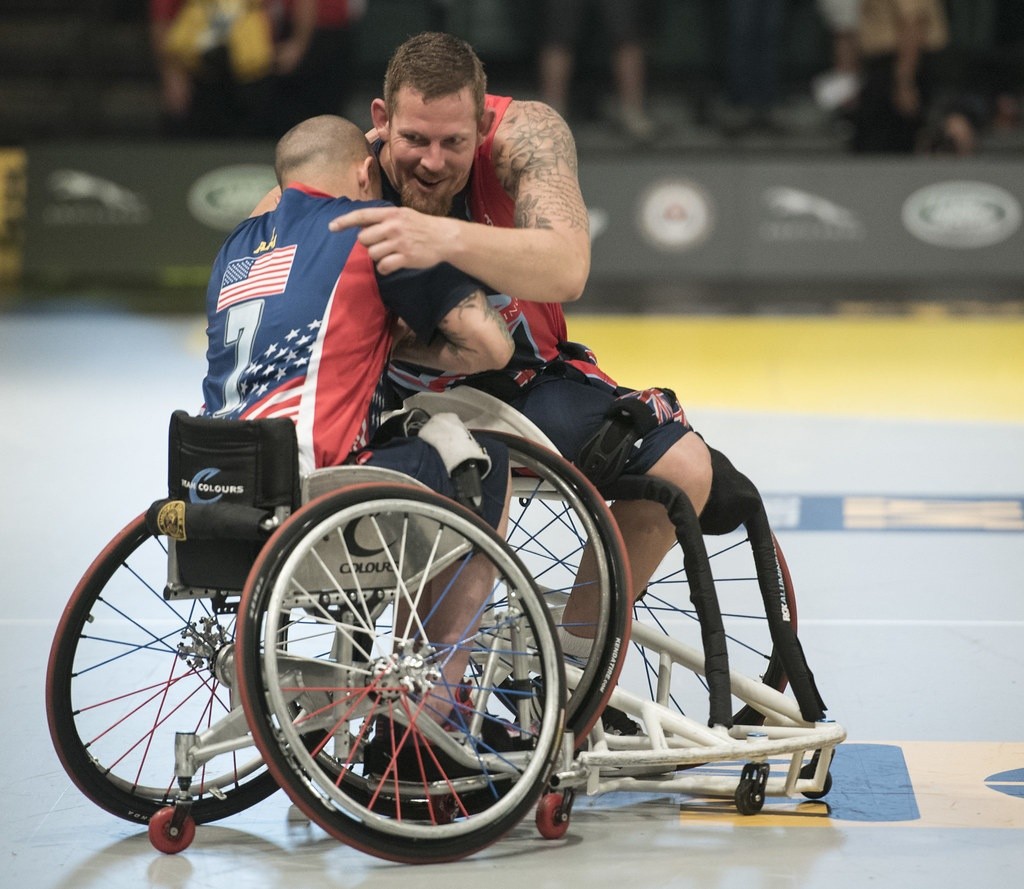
[{"x1": 43, "y1": 410, "x2": 848, "y2": 857}]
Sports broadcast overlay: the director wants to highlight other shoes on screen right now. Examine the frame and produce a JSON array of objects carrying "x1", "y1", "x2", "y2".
[
  {"x1": 362, "y1": 741, "x2": 493, "y2": 783},
  {"x1": 604, "y1": 94, "x2": 662, "y2": 142},
  {"x1": 438, "y1": 674, "x2": 535, "y2": 756},
  {"x1": 770, "y1": 91, "x2": 827, "y2": 137},
  {"x1": 529, "y1": 675, "x2": 649, "y2": 771},
  {"x1": 696, "y1": 89, "x2": 758, "y2": 139}
]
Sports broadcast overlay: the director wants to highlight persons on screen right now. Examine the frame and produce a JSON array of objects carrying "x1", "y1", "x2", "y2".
[
  {"x1": 0, "y1": 0, "x2": 991, "y2": 154},
  {"x1": 196, "y1": 34, "x2": 711, "y2": 779}
]
